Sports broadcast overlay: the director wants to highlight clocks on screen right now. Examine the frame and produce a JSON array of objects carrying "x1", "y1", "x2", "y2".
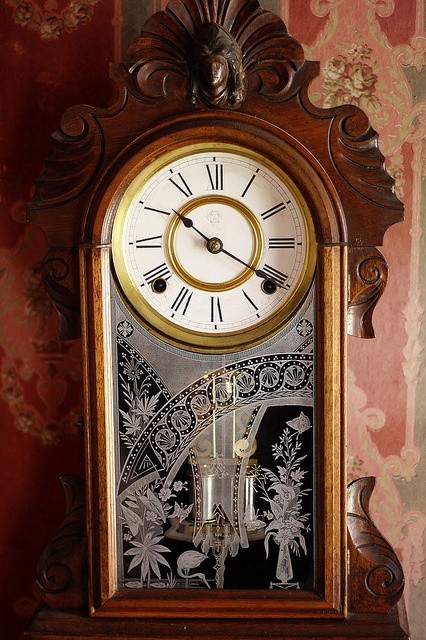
[{"x1": 80, "y1": 2, "x2": 404, "y2": 619}]
